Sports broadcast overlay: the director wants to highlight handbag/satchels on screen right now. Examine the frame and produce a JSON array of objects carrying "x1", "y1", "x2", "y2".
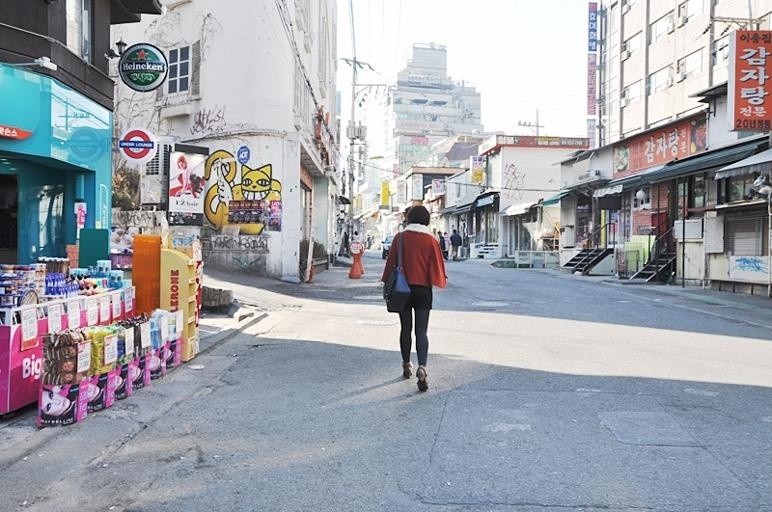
[{"x1": 383, "y1": 268, "x2": 413, "y2": 312}]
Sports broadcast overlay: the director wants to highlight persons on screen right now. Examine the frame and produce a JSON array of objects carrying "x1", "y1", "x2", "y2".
[
  {"x1": 433, "y1": 229, "x2": 462, "y2": 261},
  {"x1": 381, "y1": 205, "x2": 446, "y2": 390},
  {"x1": 344, "y1": 230, "x2": 375, "y2": 254},
  {"x1": 175, "y1": 155, "x2": 205, "y2": 198}
]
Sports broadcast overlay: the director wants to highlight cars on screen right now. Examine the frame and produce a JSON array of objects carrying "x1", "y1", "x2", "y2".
[{"x1": 381, "y1": 236, "x2": 395, "y2": 259}]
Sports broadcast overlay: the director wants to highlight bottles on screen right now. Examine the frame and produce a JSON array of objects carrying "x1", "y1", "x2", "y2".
[{"x1": 90, "y1": 266, "x2": 123, "y2": 290}]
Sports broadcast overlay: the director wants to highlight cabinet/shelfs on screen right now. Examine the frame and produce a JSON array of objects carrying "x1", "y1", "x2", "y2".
[{"x1": 159, "y1": 246, "x2": 197, "y2": 362}]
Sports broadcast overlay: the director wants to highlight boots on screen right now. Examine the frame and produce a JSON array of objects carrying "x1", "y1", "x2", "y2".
[
  {"x1": 404, "y1": 361, "x2": 412, "y2": 379},
  {"x1": 416, "y1": 365, "x2": 428, "y2": 391}
]
